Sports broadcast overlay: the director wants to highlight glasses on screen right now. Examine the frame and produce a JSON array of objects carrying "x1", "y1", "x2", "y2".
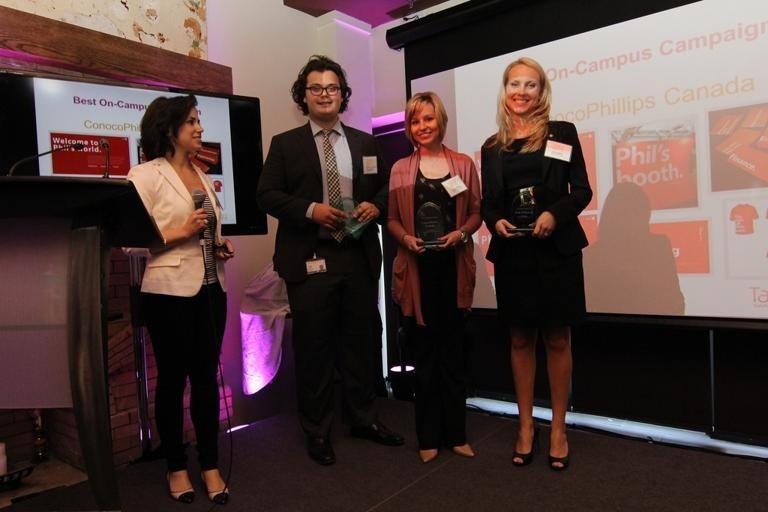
[{"x1": 307, "y1": 85, "x2": 342, "y2": 96}]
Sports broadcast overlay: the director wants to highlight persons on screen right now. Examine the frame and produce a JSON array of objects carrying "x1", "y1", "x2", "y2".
[
  {"x1": 388, "y1": 91, "x2": 480, "y2": 464},
  {"x1": 260, "y1": 56, "x2": 406, "y2": 464},
  {"x1": 481, "y1": 57, "x2": 593, "y2": 469},
  {"x1": 121, "y1": 96, "x2": 235, "y2": 505}
]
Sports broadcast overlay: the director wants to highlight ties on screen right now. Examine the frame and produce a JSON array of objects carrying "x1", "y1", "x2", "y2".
[{"x1": 321, "y1": 129, "x2": 343, "y2": 213}]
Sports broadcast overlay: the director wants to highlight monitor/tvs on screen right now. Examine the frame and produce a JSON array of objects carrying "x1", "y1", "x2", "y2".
[{"x1": 0, "y1": 70, "x2": 269, "y2": 235}]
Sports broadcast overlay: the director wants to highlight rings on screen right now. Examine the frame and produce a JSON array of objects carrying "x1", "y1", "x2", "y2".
[{"x1": 544, "y1": 232, "x2": 548, "y2": 235}]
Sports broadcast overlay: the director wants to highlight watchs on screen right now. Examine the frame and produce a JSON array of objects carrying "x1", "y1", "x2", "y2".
[{"x1": 459, "y1": 228, "x2": 469, "y2": 244}]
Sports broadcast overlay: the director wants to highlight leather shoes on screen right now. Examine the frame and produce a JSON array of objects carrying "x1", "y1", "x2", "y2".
[
  {"x1": 350, "y1": 423, "x2": 404, "y2": 446},
  {"x1": 453, "y1": 443, "x2": 474, "y2": 457},
  {"x1": 418, "y1": 448, "x2": 438, "y2": 462},
  {"x1": 308, "y1": 436, "x2": 335, "y2": 465}
]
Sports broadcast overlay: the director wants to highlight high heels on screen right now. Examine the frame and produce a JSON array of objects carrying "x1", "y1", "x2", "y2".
[
  {"x1": 548, "y1": 429, "x2": 570, "y2": 471},
  {"x1": 512, "y1": 427, "x2": 536, "y2": 466},
  {"x1": 201, "y1": 469, "x2": 229, "y2": 504},
  {"x1": 166, "y1": 469, "x2": 195, "y2": 503}
]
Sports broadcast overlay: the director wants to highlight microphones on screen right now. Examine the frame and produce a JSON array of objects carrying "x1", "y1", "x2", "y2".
[
  {"x1": 192, "y1": 187, "x2": 206, "y2": 245},
  {"x1": 8, "y1": 142, "x2": 84, "y2": 176},
  {"x1": 102, "y1": 138, "x2": 110, "y2": 178}
]
